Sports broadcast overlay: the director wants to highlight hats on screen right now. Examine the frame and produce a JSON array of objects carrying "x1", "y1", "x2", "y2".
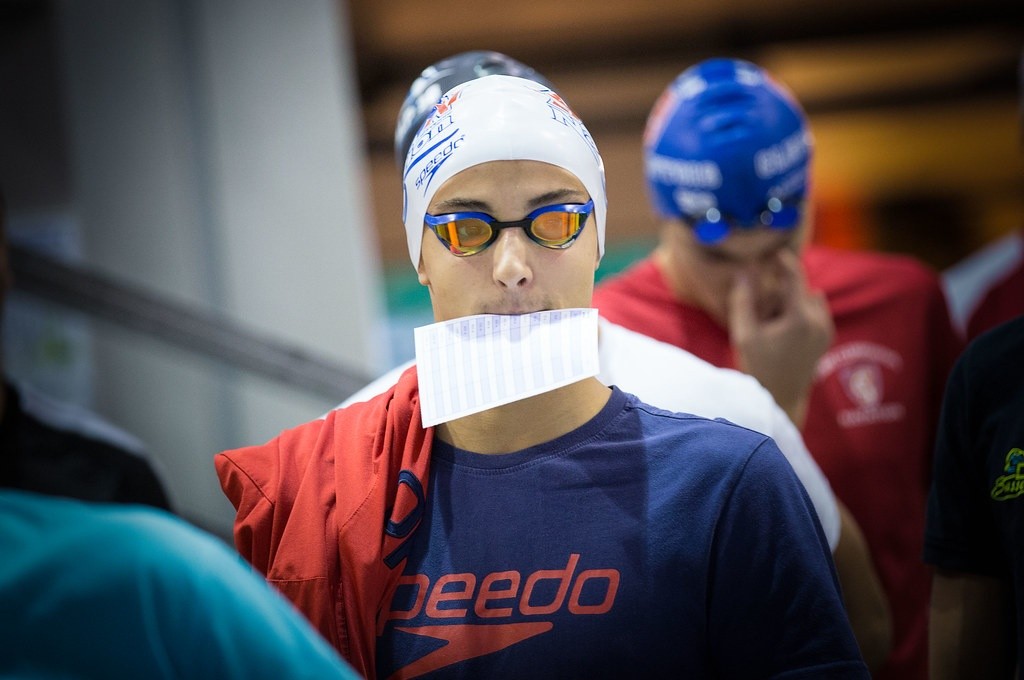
[
  {"x1": 641, "y1": 59, "x2": 810, "y2": 227},
  {"x1": 393, "y1": 51, "x2": 547, "y2": 156},
  {"x1": 402, "y1": 76, "x2": 608, "y2": 275}
]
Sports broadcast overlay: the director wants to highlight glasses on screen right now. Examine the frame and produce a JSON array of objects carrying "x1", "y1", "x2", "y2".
[
  {"x1": 674, "y1": 194, "x2": 803, "y2": 244},
  {"x1": 424, "y1": 198, "x2": 596, "y2": 259}
]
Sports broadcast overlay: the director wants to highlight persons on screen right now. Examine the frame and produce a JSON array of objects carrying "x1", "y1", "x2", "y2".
[
  {"x1": 214, "y1": 50, "x2": 1024, "y2": 680},
  {"x1": 0, "y1": 373, "x2": 362, "y2": 680}
]
